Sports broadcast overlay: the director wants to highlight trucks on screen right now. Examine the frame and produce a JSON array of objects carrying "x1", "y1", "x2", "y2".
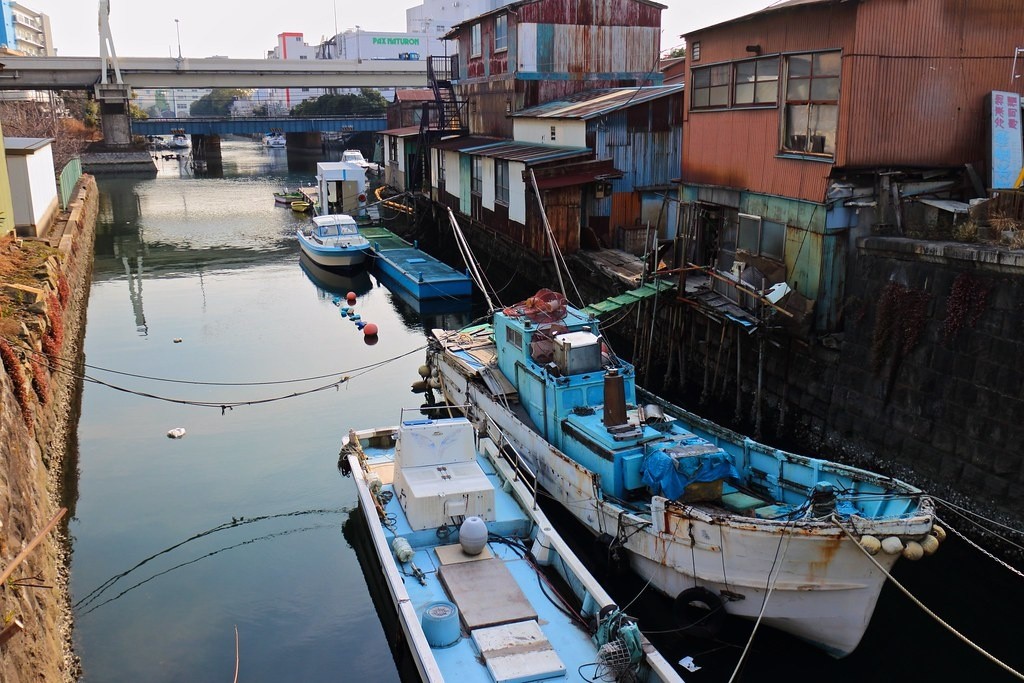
[{"x1": 371, "y1": 51, "x2": 421, "y2": 60}]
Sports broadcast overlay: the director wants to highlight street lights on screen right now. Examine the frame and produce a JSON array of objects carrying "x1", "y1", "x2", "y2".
[
  {"x1": 174, "y1": 19, "x2": 182, "y2": 58},
  {"x1": 355, "y1": 25, "x2": 361, "y2": 60}
]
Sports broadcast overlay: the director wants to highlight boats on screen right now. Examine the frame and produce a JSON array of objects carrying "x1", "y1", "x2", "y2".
[
  {"x1": 340, "y1": 404, "x2": 683, "y2": 683},
  {"x1": 291, "y1": 200, "x2": 310, "y2": 213},
  {"x1": 272, "y1": 188, "x2": 304, "y2": 204},
  {"x1": 296, "y1": 214, "x2": 372, "y2": 266},
  {"x1": 262, "y1": 132, "x2": 287, "y2": 148},
  {"x1": 148, "y1": 134, "x2": 191, "y2": 149},
  {"x1": 299, "y1": 250, "x2": 368, "y2": 298},
  {"x1": 425, "y1": 164, "x2": 947, "y2": 658}
]
